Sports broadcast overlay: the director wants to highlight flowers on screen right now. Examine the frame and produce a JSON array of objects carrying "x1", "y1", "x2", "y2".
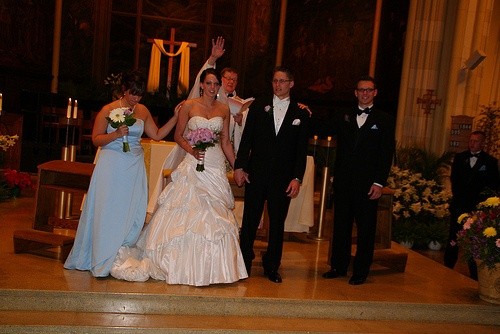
[
  {"x1": 0, "y1": 167, "x2": 32, "y2": 201},
  {"x1": 0, "y1": 133, "x2": 19, "y2": 166},
  {"x1": 264, "y1": 105, "x2": 274, "y2": 115},
  {"x1": 388, "y1": 141, "x2": 451, "y2": 244},
  {"x1": 474, "y1": 101, "x2": 500, "y2": 162},
  {"x1": 104, "y1": 72, "x2": 124, "y2": 91},
  {"x1": 185, "y1": 124, "x2": 225, "y2": 172},
  {"x1": 451, "y1": 197, "x2": 500, "y2": 273},
  {"x1": 104, "y1": 107, "x2": 137, "y2": 152}
]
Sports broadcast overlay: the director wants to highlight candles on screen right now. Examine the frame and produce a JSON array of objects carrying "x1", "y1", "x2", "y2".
[
  {"x1": 0, "y1": 93, "x2": 2, "y2": 111},
  {"x1": 327, "y1": 136, "x2": 331, "y2": 150},
  {"x1": 73, "y1": 100, "x2": 77, "y2": 119},
  {"x1": 67, "y1": 98, "x2": 72, "y2": 118}
]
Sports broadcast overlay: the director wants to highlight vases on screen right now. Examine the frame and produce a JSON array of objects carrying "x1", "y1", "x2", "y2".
[
  {"x1": 400, "y1": 239, "x2": 414, "y2": 248},
  {"x1": 474, "y1": 259, "x2": 500, "y2": 304},
  {"x1": 429, "y1": 241, "x2": 442, "y2": 251}
]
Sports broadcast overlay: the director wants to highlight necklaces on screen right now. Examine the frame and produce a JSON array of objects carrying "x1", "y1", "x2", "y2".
[{"x1": 119, "y1": 96, "x2": 136, "y2": 110}]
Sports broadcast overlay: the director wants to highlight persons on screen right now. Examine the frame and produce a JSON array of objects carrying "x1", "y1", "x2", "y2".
[
  {"x1": 444, "y1": 131, "x2": 500, "y2": 282},
  {"x1": 63, "y1": 70, "x2": 186, "y2": 278},
  {"x1": 233, "y1": 66, "x2": 311, "y2": 283},
  {"x1": 195, "y1": 36, "x2": 249, "y2": 171},
  {"x1": 298, "y1": 76, "x2": 396, "y2": 285},
  {"x1": 112, "y1": 68, "x2": 249, "y2": 285}
]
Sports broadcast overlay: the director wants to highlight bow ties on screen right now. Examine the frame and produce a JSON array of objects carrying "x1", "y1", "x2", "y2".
[
  {"x1": 272, "y1": 98, "x2": 289, "y2": 110},
  {"x1": 227, "y1": 93, "x2": 233, "y2": 97},
  {"x1": 357, "y1": 106, "x2": 371, "y2": 116},
  {"x1": 469, "y1": 153, "x2": 479, "y2": 157}
]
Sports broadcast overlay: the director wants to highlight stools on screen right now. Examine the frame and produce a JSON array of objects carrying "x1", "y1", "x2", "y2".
[{"x1": 40, "y1": 105, "x2": 98, "y2": 163}]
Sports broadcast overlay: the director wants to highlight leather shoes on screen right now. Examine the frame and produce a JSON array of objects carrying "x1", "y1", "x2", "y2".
[
  {"x1": 348, "y1": 273, "x2": 368, "y2": 284},
  {"x1": 264, "y1": 268, "x2": 282, "y2": 282},
  {"x1": 323, "y1": 269, "x2": 348, "y2": 279}
]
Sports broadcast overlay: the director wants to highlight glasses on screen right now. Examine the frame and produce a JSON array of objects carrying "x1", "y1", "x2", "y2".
[
  {"x1": 222, "y1": 76, "x2": 237, "y2": 83},
  {"x1": 272, "y1": 79, "x2": 292, "y2": 86},
  {"x1": 357, "y1": 87, "x2": 374, "y2": 95}
]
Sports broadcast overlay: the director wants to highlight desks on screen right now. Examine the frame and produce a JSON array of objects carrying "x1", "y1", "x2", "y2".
[
  {"x1": 32, "y1": 160, "x2": 96, "y2": 232},
  {"x1": 140, "y1": 139, "x2": 315, "y2": 242}
]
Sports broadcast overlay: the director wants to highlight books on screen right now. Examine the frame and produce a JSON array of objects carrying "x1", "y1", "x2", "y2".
[{"x1": 227, "y1": 95, "x2": 253, "y2": 115}]
